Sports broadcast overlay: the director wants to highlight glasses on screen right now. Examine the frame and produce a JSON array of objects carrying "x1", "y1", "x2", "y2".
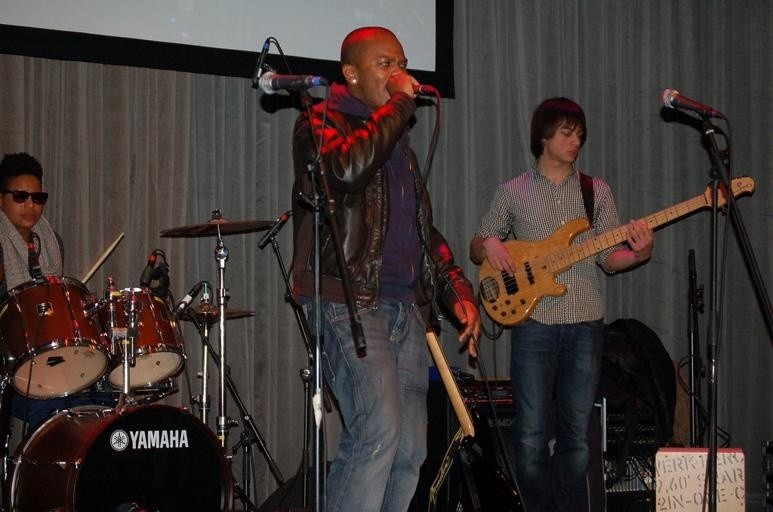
[{"x1": 7, "y1": 189, "x2": 48, "y2": 205}]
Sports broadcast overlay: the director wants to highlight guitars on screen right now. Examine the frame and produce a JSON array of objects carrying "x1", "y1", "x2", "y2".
[{"x1": 478, "y1": 177, "x2": 755, "y2": 326}]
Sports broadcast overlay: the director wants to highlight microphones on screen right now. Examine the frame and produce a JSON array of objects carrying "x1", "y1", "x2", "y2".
[
  {"x1": 140, "y1": 251, "x2": 157, "y2": 286},
  {"x1": 413, "y1": 85, "x2": 441, "y2": 102},
  {"x1": 176, "y1": 282, "x2": 203, "y2": 314},
  {"x1": 258, "y1": 71, "x2": 327, "y2": 95},
  {"x1": 659, "y1": 88, "x2": 725, "y2": 119}
]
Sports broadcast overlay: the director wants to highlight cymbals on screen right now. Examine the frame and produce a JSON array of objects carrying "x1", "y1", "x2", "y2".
[
  {"x1": 172, "y1": 306, "x2": 255, "y2": 324},
  {"x1": 158, "y1": 219, "x2": 278, "y2": 238}
]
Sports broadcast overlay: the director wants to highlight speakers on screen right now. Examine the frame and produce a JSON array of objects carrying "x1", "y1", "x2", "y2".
[{"x1": 424, "y1": 380, "x2": 518, "y2": 511}]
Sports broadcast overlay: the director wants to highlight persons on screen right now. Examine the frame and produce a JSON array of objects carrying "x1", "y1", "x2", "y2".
[
  {"x1": 0, "y1": 153, "x2": 66, "y2": 306},
  {"x1": 468, "y1": 94, "x2": 655, "y2": 512},
  {"x1": 286, "y1": 21, "x2": 482, "y2": 512}
]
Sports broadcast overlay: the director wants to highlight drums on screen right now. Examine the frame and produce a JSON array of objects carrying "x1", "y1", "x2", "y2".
[
  {"x1": 0, "y1": 275, "x2": 113, "y2": 401},
  {"x1": 2, "y1": 404, "x2": 235, "y2": 512},
  {"x1": 95, "y1": 286, "x2": 187, "y2": 390}
]
